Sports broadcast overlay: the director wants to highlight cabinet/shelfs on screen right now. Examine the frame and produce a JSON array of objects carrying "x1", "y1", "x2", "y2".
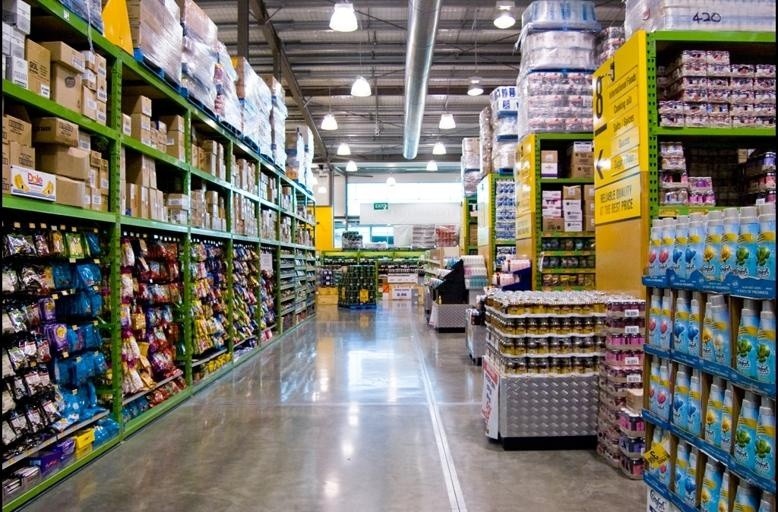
[
  {"x1": 0, "y1": 0, "x2": 316, "y2": 512},
  {"x1": 591, "y1": 28, "x2": 778, "y2": 301},
  {"x1": 419, "y1": 173, "x2": 533, "y2": 303},
  {"x1": 514, "y1": 133, "x2": 595, "y2": 291}
]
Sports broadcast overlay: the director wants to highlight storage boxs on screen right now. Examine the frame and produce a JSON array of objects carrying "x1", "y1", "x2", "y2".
[
  {"x1": 541, "y1": 140, "x2": 596, "y2": 234},
  {"x1": 0, "y1": 0, "x2": 316, "y2": 245},
  {"x1": 626, "y1": 387, "x2": 646, "y2": 414}
]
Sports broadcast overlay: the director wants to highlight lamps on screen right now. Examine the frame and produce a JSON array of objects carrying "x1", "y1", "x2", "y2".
[{"x1": 320, "y1": 0, "x2": 515, "y2": 172}]
